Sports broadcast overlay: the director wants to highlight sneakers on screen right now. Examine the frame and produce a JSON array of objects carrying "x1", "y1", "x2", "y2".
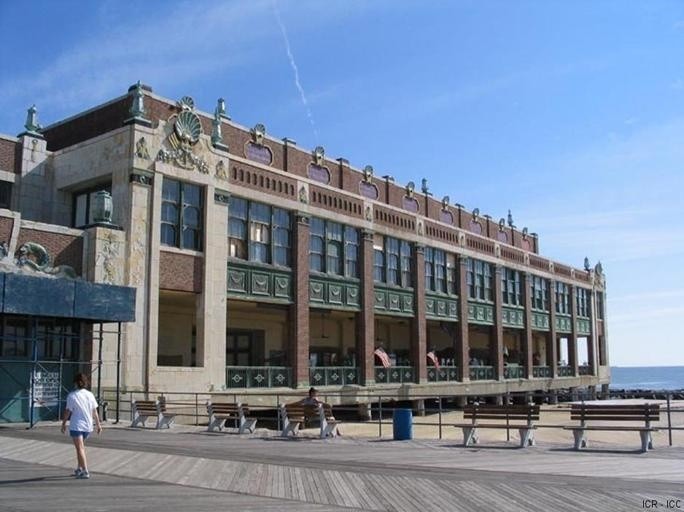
[
  {"x1": 75, "y1": 471, "x2": 89, "y2": 479},
  {"x1": 70, "y1": 469, "x2": 83, "y2": 477}
]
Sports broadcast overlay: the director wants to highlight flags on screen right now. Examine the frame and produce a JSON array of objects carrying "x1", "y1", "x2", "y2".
[
  {"x1": 426, "y1": 349, "x2": 440, "y2": 370},
  {"x1": 374, "y1": 346, "x2": 392, "y2": 368}
]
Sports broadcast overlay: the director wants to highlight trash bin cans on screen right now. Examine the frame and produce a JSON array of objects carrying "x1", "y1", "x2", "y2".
[
  {"x1": 393, "y1": 408, "x2": 414, "y2": 440},
  {"x1": 503, "y1": 363, "x2": 508, "y2": 368}
]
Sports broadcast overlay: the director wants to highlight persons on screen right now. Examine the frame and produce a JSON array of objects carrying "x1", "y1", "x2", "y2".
[
  {"x1": 61, "y1": 372, "x2": 101, "y2": 480},
  {"x1": 302, "y1": 386, "x2": 344, "y2": 438}
]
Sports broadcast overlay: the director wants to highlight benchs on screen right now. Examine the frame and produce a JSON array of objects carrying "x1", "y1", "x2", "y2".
[
  {"x1": 564, "y1": 403, "x2": 660, "y2": 453},
  {"x1": 453, "y1": 404, "x2": 542, "y2": 452},
  {"x1": 281, "y1": 405, "x2": 343, "y2": 441},
  {"x1": 205, "y1": 402, "x2": 258, "y2": 435},
  {"x1": 132, "y1": 400, "x2": 176, "y2": 429}
]
